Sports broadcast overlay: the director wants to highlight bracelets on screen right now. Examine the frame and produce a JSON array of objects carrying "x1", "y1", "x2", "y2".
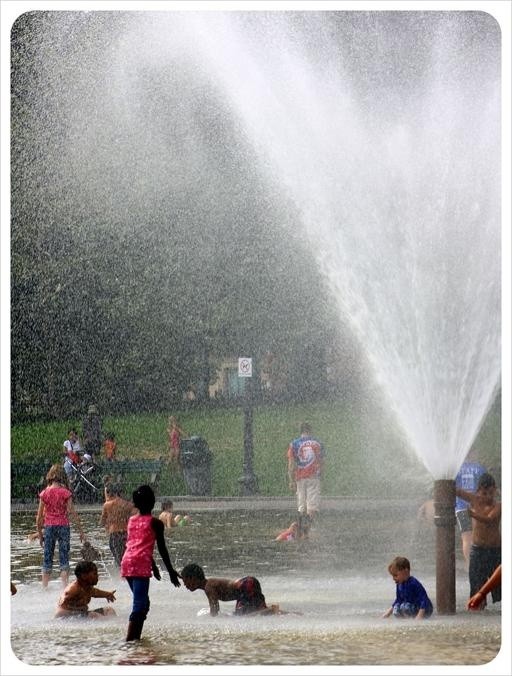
[{"x1": 478, "y1": 591, "x2": 484, "y2": 595}]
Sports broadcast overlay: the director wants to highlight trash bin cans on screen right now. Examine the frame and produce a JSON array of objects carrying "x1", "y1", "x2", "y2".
[{"x1": 181, "y1": 437, "x2": 214, "y2": 494}]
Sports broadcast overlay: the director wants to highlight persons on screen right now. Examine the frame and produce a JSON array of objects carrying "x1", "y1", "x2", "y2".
[
  {"x1": 12, "y1": 582, "x2": 17, "y2": 596},
  {"x1": 101, "y1": 482, "x2": 183, "y2": 641},
  {"x1": 37, "y1": 465, "x2": 83, "y2": 590},
  {"x1": 54, "y1": 561, "x2": 116, "y2": 617},
  {"x1": 382, "y1": 557, "x2": 433, "y2": 621},
  {"x1": 454, "y1": 460, "x2": 490, "y2": 573},
  {"x1": 63, "y1": 405, "x2": 117, "y2": 496},
  {"x1": 277, "y1": 424, "x2": 327, "y2": 542},
  {"x1": 159, "y1": 499, "x2": 192, "y2": 528},
  {"x1": 455, "y1": 473, "x2": 500, "y2": 607},
  {"x1": 416, "y1": 489, "x2": 437, "y2": 538},
  {"x1": 167, "y1": 416, "x2": 189, "y2": 461},
  {"x1": 181, "y1": 564, "x2": 280, "y2": 615},
  {"x1": 465, "y1": 564, "x2": 501, "y2": 614}
]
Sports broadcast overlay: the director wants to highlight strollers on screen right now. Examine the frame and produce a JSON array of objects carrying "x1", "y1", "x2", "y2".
[{"x1": 65, "y1": 457, "x2": 104, "y2": 504}]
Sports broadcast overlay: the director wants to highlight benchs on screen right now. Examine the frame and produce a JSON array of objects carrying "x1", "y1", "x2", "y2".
[{"x1": 87, "y1": 454, "x2": 162, "y2": 500}]
